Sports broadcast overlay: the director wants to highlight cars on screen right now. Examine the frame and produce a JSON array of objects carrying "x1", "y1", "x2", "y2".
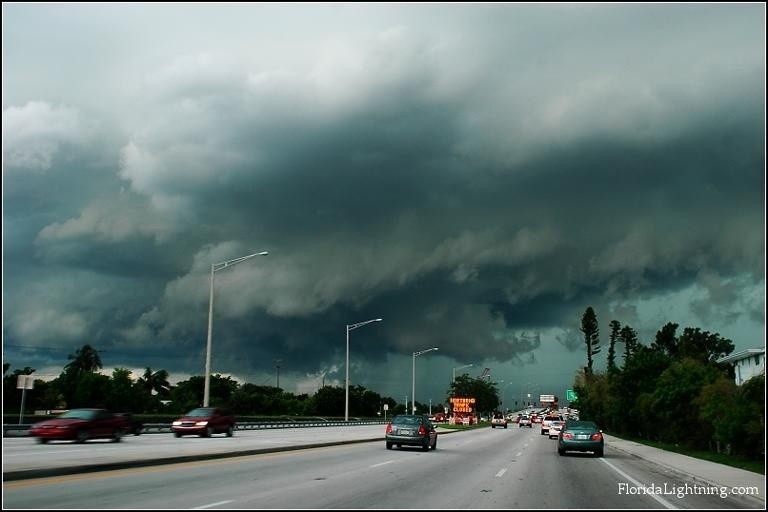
[
  {"x1": 550, "y1": 422, "x2": 565, "y2": 438},
  {"x1": 532, "y1": 408, "x2": 551, "y2": 422},
  {"x1": 558, "y1": 405, "x2": 579, "y2": 420},
  {"x1": 539, "y1": 415, "x2": 560, "y2": 436},
  {"x1": 31, "y1": 407, "x2": 128, "y2": 443},
  {"x1": 117, "y1": 411, "x2": 141, "y2": 436},
  {"x1": 491, "y1": 413, "x2": 507, "y2": 431},
  {"x1": 385, "y1": 413, "x2": 436, "y2": 452},
  {"x1": 171, "y1": 408, "x2": 234, "y2": 438},
  {"x1": 558, "y1": 420, "x2": 603, "y2": 456},
  {"x1": 518, "y1": 417, "x2": 532, "y2": 427},
  {"x1": 434, "y1": 413, "x2": 447, "y2": 422}
]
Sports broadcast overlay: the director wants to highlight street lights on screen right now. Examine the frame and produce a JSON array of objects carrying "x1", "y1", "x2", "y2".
[
  {"x1": 203, "y1": 251, "x2": 271, "y2": 410},
  {"x1": 344, "y1": 318, "x2": 380, "y2": 420},
  {"x1": 452, "y1": 364, "x2": 472, "y2": 397},
  {"x1": 411, "y1": 348, "x2": 436, "y2": 418},
  {"x1": 500, "y1": 382, "x2": 511, "y2": 411}
]
